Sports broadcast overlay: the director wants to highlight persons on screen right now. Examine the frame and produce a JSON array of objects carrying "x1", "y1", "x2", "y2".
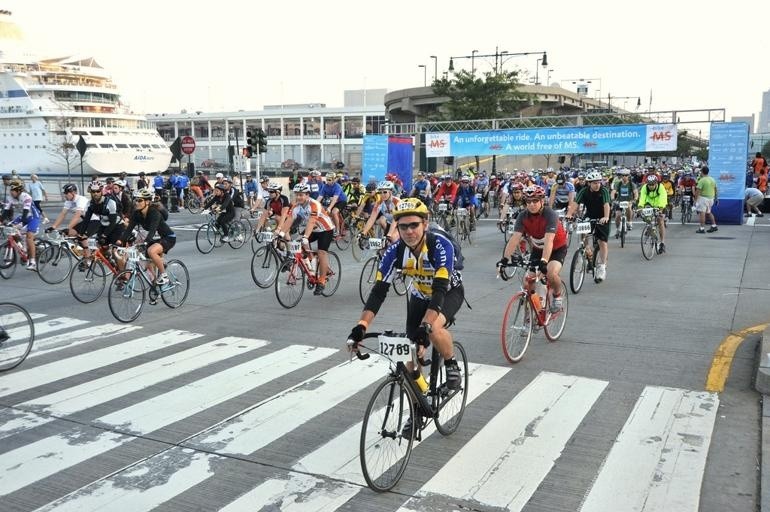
[{"x1": 347, "y1": 198, "x2": 465, "y2": 391}]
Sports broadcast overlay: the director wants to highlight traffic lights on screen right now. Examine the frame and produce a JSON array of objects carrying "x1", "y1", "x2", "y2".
[
  {"x1": 246, "y1": 128, "x2": 258, "y2": 153},
  {"x1": 242, "y1": 148, "x2": 252, "y2": 158},
  {"x1": 260, "y1": 127, "x2": 268, "y2": 154}
]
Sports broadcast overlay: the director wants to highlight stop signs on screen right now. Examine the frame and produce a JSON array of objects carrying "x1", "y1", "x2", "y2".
[{"x1": 179, "y1": 135, "x2": 195, "y2": 155}]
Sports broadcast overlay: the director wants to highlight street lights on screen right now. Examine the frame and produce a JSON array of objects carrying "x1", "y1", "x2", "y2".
[
  {"x1": 447, "y1": 51, "x2": 551, "y2": 76},
  {"x1": 578, "y1": 90, "x2": 642, "y2": 123}
]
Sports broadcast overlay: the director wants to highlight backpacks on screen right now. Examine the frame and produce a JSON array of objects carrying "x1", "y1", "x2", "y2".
[{"x1": 190, "y1": 175, "x2": 203, "y2": 186}]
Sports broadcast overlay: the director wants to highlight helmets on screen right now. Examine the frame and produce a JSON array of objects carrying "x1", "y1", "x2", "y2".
[
  {"x1": 645, "y1": 174, "x2": 657, "y2": 183},
  {"x1": 585, "y1": 171, "x2": 603, "y2": 182},
  {"x1": 197, "y1": 171, "x2": 203, "y2": 175},
  {"x1": 222, "y1": 176, "x2": 233, "y2": 184},
  {"x1": 366, "y1": 183, "x2": 377, "y2": 191},
  {"x1": 416, "y1": 168, "x2": 502, "y2": 182},
  {"x1": 266, "y1": 182, "x2": 282, "y2": 194},
  {"x1": 502, "y1": 168, "x2": 585, "y2": 190},
  {"x1": 259, "y1": 175, "x2": 270, "y2": 183},
  {"x1": 91, "y1": 171, "x2": 127, "y2": 189},
  {"x1": 293, "y1": 183, "x2": 311, "y2": 193},
  {"x1": 215, "y1": 172, "x2": 223, "y2": 179},
  {"x1": 140, "y1": 172, "x2": 145, "y2": 177},
  {"x1": 377, "y1": 181, "x2": 394, "y2": 191},
  {"x1": 385, "y1": 173, "x2": 398, "y2": 182},
  {"x1": 154, "y1": 169, "x2": 187, "y2": 176},
  {"x1": 308, "y1": 170, "x2": 321, "y2": 177},
  {"x1": 10, "y1": 179, "x2": 25, "y2": 191},
  {"x1": 392, "y1": 196, "x2": 429, "y2": 219},
  {"x1": 617, "y1": 168, "x2": 631, "y2": 177},
  {"x1": 326, "y1": 169, "x2": 360, "y2": 183},
  {"x1": 523, "y1": 184, "x2": 545, "y2": 199},
  {"x1": 63, "y1": 184, "x2": 77, "y2": 193},
  {"x1": 215, "y1": 184, "x2": 225, "y2": 191},
  {"x1": 131, "y1": 187, "x2": 151, "y2": 199}
]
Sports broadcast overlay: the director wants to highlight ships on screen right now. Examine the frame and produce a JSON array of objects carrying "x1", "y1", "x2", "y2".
[{"x1": 0, "y1": 37, "x2": 175, "y2": 179}]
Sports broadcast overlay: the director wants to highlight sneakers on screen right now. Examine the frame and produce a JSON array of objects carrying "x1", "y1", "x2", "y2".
[
  {"x1": 595, "y1": 264, "x2": 606, "y2": 282},
  {"x1": 519, "y1": 320, "x2": 532, "y2": 337},
  {"x1": 401, "y1": 416, "x2": 419, "y2": 439},
  {"x1": 695, "y1": 227, "x2": 705, "y2": 233},
  {"x1": 745, "y1": 213, "x2": 752, "y2": 217},
  {"x1": 154, "y1": 273, "x2": 170, "y2": 285},
  {"x1": 149, "y1": 292, "x2": 161, "y2": 305},
  {"x1": 756, "y1": 214, "x2": 764, "y2": 217},
  {"x1": 314, "y1": 281, "x2": 324, "y2": 296},
  {"x1": 26, "y1": 262, "x2": 38, "y2": 271},
  {"x1": 707, "y1": 225, "x2": 718, "y2": 233},
  {"x1": 221, "y1": 235, "x2": 229, "y2": 241},
  {"x1": 444, "y1": 358, "x2": 464, "y2": 388},
  {"x1": 552, "y1": 293, "x2": 563, "y2": 314}
]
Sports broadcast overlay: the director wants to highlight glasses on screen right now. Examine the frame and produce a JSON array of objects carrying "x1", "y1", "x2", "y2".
[
  {"x1": 268, "y1": 190, "x2": 279, "y2": 193},
  {"x1": 135, "y1": 199, "x2": 144, "y2": 203},
  {"x1": 526, "y1": 198, "x2": 539, "y2": 203},
  {"x1": 63, "y1": 191, "x2": 72, "y2": 194},
  {"x1": 91, "y1": 191, "x2": 100, "y2": 194},
  {"x1": 397, "y1": 221, "x2": 424, "y2": 231},
  {"x1": 377, "y1": 191, "x2": 390, "y2": 194}
]
